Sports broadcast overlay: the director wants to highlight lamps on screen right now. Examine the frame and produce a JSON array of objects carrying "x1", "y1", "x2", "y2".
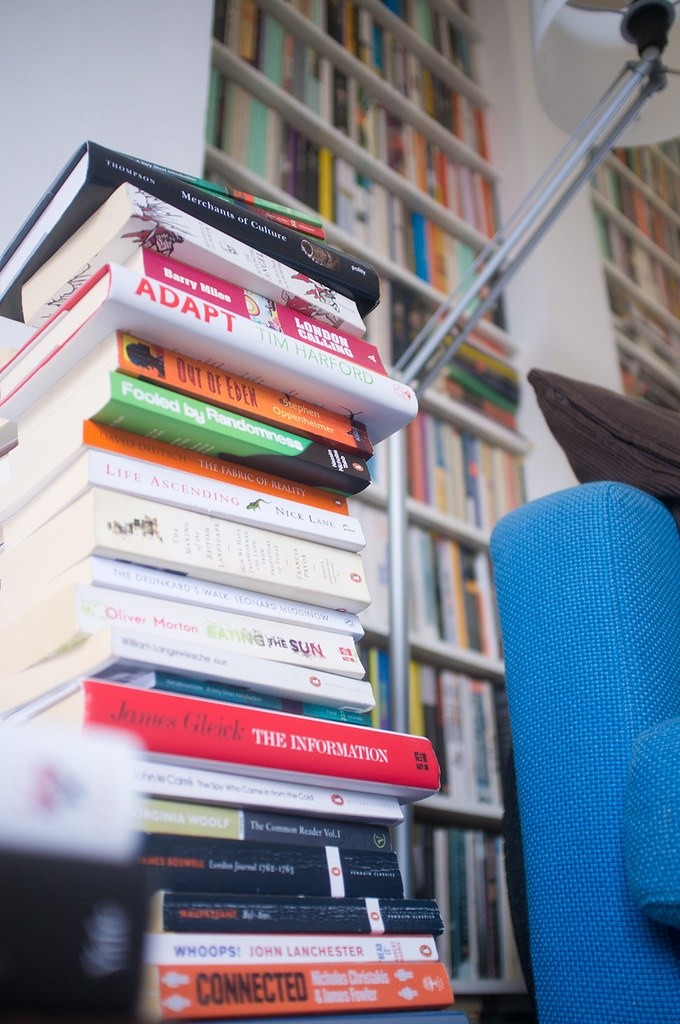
[{"x1": 392, "y1": 0, "x2": 680, "y2": 898}]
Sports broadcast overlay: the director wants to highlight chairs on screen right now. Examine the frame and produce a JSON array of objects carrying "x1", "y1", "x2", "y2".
[{"x1": 488, "y1": 480, "x2": 680, "y2": 1024}]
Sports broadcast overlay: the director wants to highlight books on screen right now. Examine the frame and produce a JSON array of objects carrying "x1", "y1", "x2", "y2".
[
  {"x1": 593, "y1": 136, "x2": 680, "y2": 406},
  {"x1": 200, "y1": 0, "x2": 529, "y2": 980},
  {"x1": 0, "y1": 139, "x2": 453, "y2": 1024}
]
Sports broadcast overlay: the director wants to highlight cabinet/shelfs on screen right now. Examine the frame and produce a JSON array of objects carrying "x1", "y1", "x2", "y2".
[{"x1": 200, "y1": 0, "x2": 680, "y2": 1023}]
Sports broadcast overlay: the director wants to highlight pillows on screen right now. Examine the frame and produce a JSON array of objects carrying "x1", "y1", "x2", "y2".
[{"x1": 526, "y1": 367, "x2": 679, "y2": 508}]
